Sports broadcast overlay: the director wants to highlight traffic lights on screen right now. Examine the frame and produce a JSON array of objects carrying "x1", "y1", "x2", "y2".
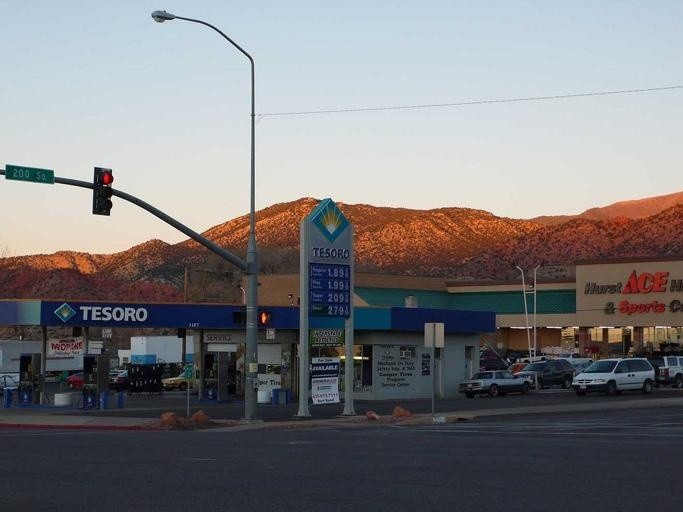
[{"x1": 90, "y1": 166, "x2": 112, "y2": 219}]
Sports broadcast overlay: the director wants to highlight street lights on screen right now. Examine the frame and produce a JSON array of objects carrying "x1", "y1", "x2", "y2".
[
  {"x1": 235, "y1": 281, "x2": 262, "y2": 305},
  {"x1": 151, "y1": 8, "x2": 258, "y2": 420},
  {"x1": 508, "y1": 258, "x2": 547, "y2": 363}
]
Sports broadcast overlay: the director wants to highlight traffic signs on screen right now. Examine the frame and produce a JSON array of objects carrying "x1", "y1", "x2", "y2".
[{"x1": 5, "y1": 165, "x2": 55, "y2": 184}]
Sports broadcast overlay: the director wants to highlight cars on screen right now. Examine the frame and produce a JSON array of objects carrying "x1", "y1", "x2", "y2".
[
  {"x1": 66, "y1": 364, "x2": 218, "y2": 392},
  {"x1": 458, "y1": 370, "x2": 535, "y2": 400},
  {"x1": 480, "y1": 349, "x2": 682, "y2": 396}
]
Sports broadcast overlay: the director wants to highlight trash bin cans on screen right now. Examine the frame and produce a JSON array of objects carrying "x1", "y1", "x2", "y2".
[
  {"x1": 100, "y1": 391, "x2": 126, "y2": 408},
  {"x1": 272, "y1": 388, "x2": 292, "y2": 406}
]
